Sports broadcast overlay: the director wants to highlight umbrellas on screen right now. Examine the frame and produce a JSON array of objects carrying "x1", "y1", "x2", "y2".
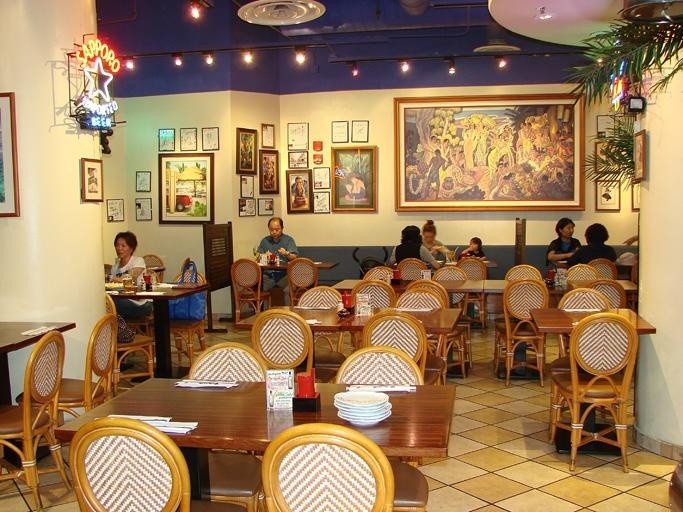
[{"x1": 177, "y1": 167, "x2": 205, "y2": 196}]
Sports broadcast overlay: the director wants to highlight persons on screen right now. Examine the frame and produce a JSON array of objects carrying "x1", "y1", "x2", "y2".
[
  {"x1": 545, "y1": 218, "x2": 582, "y2": 266},
  {"x1": 460, "y1": 236, "x2": 486, "y2": 258},
  {"x1": 420, "y1": 219, "x2": 451, "y2": 261},
  {"x1": 389, "y1": 225, "x2": 440, "y2": 269},
  {"x1": 346, "y1": 176, "x2": 366, "y2": 199},
  {"x1": 256, "y1": 217, "x2": 299, "y2": 309},
  {"x1": 422, "y1": 117, "x2": 559, "y2": 199},
  {"x1": 295, "y1": 177, "x2": 305, "y2": 197},
  {"x1": 110, "y1": 231, "x2": 152, "y2": 319},
  {"x1": 566, "y1": 222, "x2": 617, "y2": 270}
]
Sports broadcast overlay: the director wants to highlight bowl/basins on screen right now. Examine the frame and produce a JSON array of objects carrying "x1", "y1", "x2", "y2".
[
  {"x1": 337, "y1": 313, "x2": 350, "y2": 319},
  {"x1": 545, "y1": 281, "x2": 555, "y2": 286}
]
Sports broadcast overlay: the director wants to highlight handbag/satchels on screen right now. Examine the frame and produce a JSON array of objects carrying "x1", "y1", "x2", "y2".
[
  {"x1": 116, "y1": 315, "x2": 135, "y2": 343},
  {"x1": 167, "y1": 293, "x2": 207, "y2": 321}
]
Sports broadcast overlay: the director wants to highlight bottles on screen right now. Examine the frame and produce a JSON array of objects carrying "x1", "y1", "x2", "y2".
[
  {"x1": 269, "y1": 390, "x2": 274, "y2": 408},
  {"x1": 276, "y1": 256, "x2": 280, "y2": 267},
  {"x1": 140, "y1": 278, "x2": 146, "y2": 290},
  {"x1": 287, "y1": 371, "x2": 294, "y2": 389},
  {"x1": 338, "y1": 300, "x2": 344, "y2": 313}
]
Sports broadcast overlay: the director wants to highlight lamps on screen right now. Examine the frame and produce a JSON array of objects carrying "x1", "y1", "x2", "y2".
[
  {"x1": 237, "y1": 1, "x2": 326, "y2": 26},
  {"x1": 449, "y1": 62, "x2": 456, "y2": 75}
]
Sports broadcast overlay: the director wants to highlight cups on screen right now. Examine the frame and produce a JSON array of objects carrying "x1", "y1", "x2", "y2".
[
  {"x1": 122, "y1": 273, "x2": 132, "y2": 293},
  {"x1": 450, "y1": 252, "x2": 456, "y2": 261},
  {"x1": 446, "y1": 252, "x2": 453, "y2": 263}
]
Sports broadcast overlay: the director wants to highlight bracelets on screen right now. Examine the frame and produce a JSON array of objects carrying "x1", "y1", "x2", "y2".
[{"x1": 285, "y1": 250, "x2": 290, "y2": 256}]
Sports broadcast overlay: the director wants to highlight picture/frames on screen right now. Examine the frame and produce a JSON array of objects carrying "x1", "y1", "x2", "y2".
[
  {"x1": 258, "y1": 198, "x2": 274, "y2": 216},
  {"x1": 595, "y1": 142, "x2": 620, "y2": 174},
  {"x1": 0, "y1": 92, "x2": 20, "y2": 217},
  {"x1": 288, "y1": 151, "x2": 308, "y2": 169},
  {"x1": 202, "y1": 127, "x2": 219, "y2": 151},
  {"x1": 159, "y1": 129, "x2": 175, "y2": 151},
  {"x1": 287, "y1": 123, "x2": 309, "y2": 151},
  {"x1": 597, "y1": 115, "x2": 615, "y2": 138},
  {"x1": 314, "y1": 167, "x2": 330, "y2": 189},
  {"x1": 180, "y1": 128, "x2": 197, "y2": 151},
  {"x1": 240, "y1": 175, "x2": 254, "y2": 198},
  {"x1": 632, "y1": 182, "x2": 640, "y2": 210},
  {"x1": 239, "y1": 198, "x2": 256, "y2": 217},
  {"x1": 136, "y1": 171, "x2": 151, "y2": 192},
  {"x1": 135, "y1": 198, "x2": 152, "y2": 221},
  {"x1": 331, "y1": 121, "x2": 348, "y2": 142},
  {"x1": 633, "y1": 130, "x2": 646, "y2": 180},
  {"x1": 107, "y1": 199, "x2": 124, "y2": 221},
  {"x1": 595, "y1": 180, "x2": 620, "y2": 210},
  {"x1": 236, "y1": 128, "x2": 257, "y2": 175},
  {"x1": 259, "y1": 150, "x2": 279, "y2": 194},
  {"x1": 81, "y1": 158, "x2": 103, "y2": 202},
  {"x1": 158, "y1": 153, "x2": 214, "y2": 224},
  {"x1": 314, "y1": 192, "x2": 330, "y2": 213},
  {"x1": 286, "y1": 169, "x2": 314, "y2": 214},
  {"x1": 331, "y1": 145, "x2": 379, "y2": 213},
  {"x1": 393, "y1": 93, "x2": 585, "y2": 212},
  {"x1": 261, "y1": 124, "x2": 275, "y2": 148},
  {"x1": 351, "y1": 120, "x2": 369, "y2": 142},
  {"x1": 619, "y1": 115, "x2": 636, "y2": 137}
]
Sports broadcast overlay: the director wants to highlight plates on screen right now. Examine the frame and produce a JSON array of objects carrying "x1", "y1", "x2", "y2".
[
  {"x1": 438, "y1": 261, "x2": 443, "y2": 264},
  {"x1": 332, "y1": 392, "x2": 391, "y2": 428},
  {"x1": 106, "y1": 281, "x2": 123, "y2": 291}
]
